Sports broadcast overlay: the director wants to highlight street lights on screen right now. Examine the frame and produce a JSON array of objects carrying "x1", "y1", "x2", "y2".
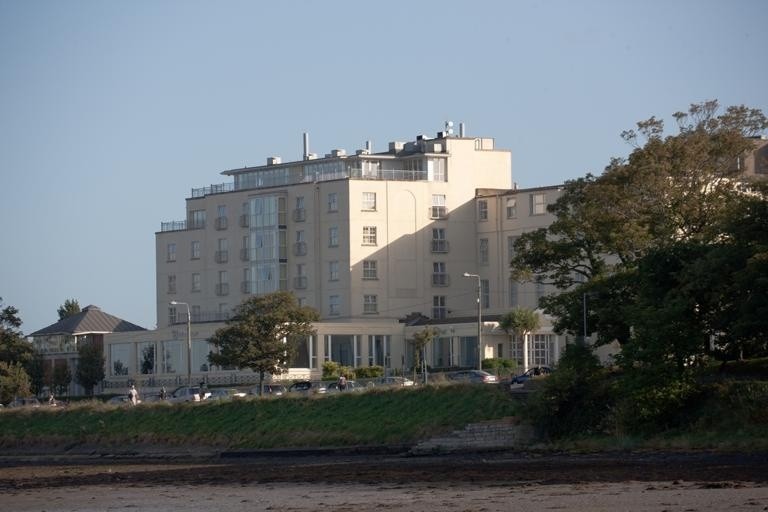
[
  {"x1": 583, "y1": 290, "x2": 601, "y2": 339},
  {"x1": 169, "y1": 301, "x2": 193, "y2": 386},
  {"x1": 463, "y1": 272, "x2": 483, "y2": 369}
]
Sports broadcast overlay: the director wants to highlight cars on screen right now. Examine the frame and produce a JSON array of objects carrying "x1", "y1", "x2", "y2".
[
  {"x1": 5, "y1": 398, "x2": 41, "y2": 410},
  {"x1": 109, "y1": 380, "x2": 327, "y2": 406},
  {"x1": 376, "y1": 375, "x2": 420, "y2": 387},
  {"x1": 447, "y1": 370, "x2": 498, "y2": 384},
  {"x1": 327, "y1": 381, "x2": 366, "y2": 392},
  {"x1": 513, "y1": 365, "x2": 552, "y2": 385}
]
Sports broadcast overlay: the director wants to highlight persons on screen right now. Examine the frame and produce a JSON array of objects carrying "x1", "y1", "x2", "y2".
[
  {"x1": 128, "y1": 383, "x2": 139, "y2": 407},
  {"x1": 47, "y1": 392, "x2": 71, "y2": 407},
  {"x1": 336, "y1": 371, "x2": 346, "y2": 392},
  {"x1": 159, "y1": 384, "x2": 167, "y2": 401},
  {"x1": 198, "y1": 381, "x2": 211, "y2": 401}
]
